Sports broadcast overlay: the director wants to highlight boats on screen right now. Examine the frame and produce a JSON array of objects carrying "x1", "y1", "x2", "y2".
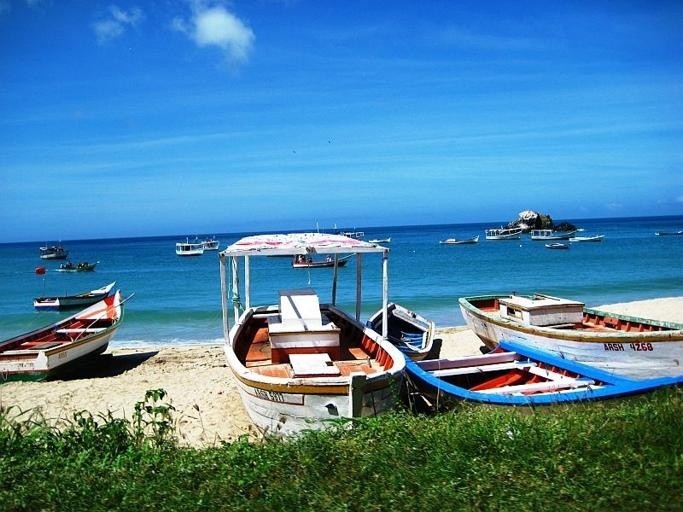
[
  {"x1": 0, "y1": 290, "x2": 122, "y2": 381},
  {"x1": 216, "y1": 231, "x2": 407, "y2": 446},
  {"x1": 38, "y1": 240, "x2": 69, "y2": 259},
  {"x1": 437, "y1": 224, "x2": 606, "y2": 250},
  {"x1": 54, "y1": 260, "x2": 99, "y2": 273},
  {"x1": 291, "y1": 218, "x2": 392, "y2": 270},
  {"x1": 653, "y1": 230, "x2": 683, "y2": 236},
  {"x1": 175, "y1": 235, "x2": 203, "y2": 256},
  {"x1": 366, "y1": 301, "x2": 437, "y2": 358},
  {"x1": 397, "y1": 337, "x2": 683, "y2": 423},
  {"x1": 30, "y1": 280, "x2": 117, "y2": 310},
  {"x1": 201, "y1": 237, "x2": 219, "y2": 252},
  {"x1": 454, "y1": 289, "x2": 682, "y2": 384}
]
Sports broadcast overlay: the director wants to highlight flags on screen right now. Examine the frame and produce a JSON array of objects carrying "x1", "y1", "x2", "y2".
[{"x1": 35, "y1": 266, "x2": 45, "y2": 275}]
